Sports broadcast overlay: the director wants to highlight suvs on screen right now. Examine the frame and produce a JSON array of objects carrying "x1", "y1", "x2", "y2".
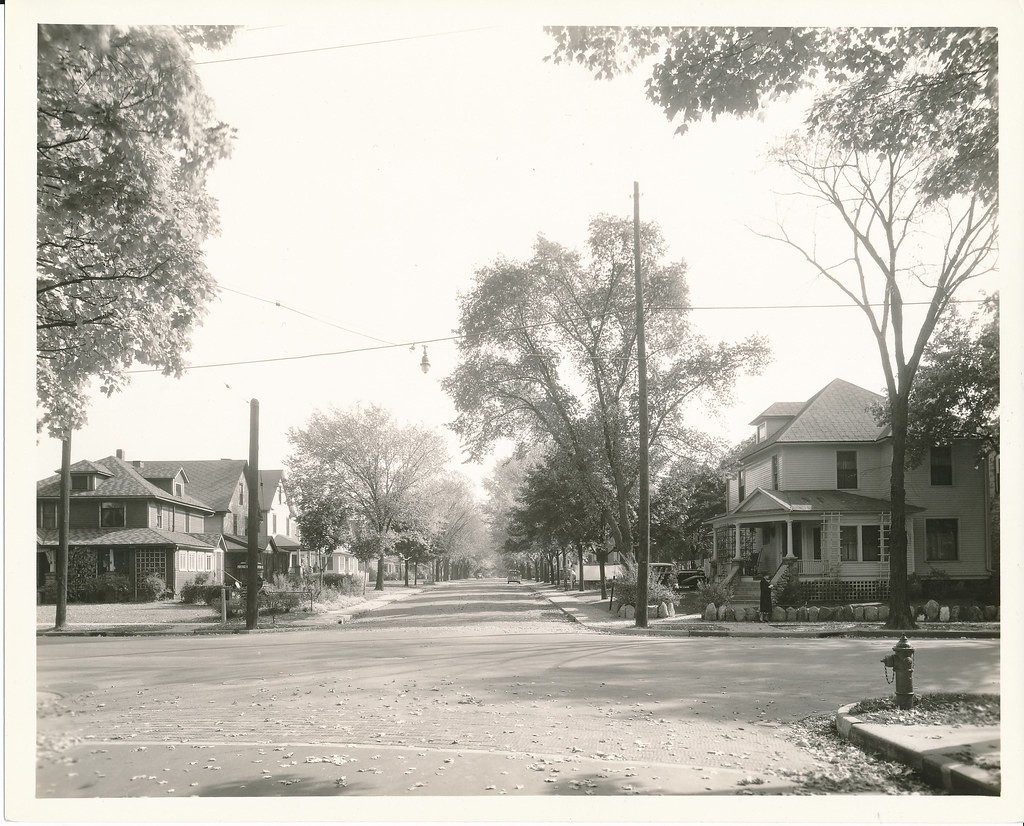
[
  {"x1": 648, "y1": 562, "x2": 677, "y2": 589},
  {"x1": 507, "y1": 570, "x2": 522, "y2": 584}
]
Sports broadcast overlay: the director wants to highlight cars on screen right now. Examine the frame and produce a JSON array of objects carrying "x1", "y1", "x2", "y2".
[
  {"x1": 677, "y1": 569, "x2": 707, "y2": 588},
  {"x1": 477, "y1": 573, "x2": 483, "y2": 579}
]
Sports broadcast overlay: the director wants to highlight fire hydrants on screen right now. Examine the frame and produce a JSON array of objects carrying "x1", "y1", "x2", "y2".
[{"x1": 878, "y1": 634, "x2": 916, "y2": 711}]
